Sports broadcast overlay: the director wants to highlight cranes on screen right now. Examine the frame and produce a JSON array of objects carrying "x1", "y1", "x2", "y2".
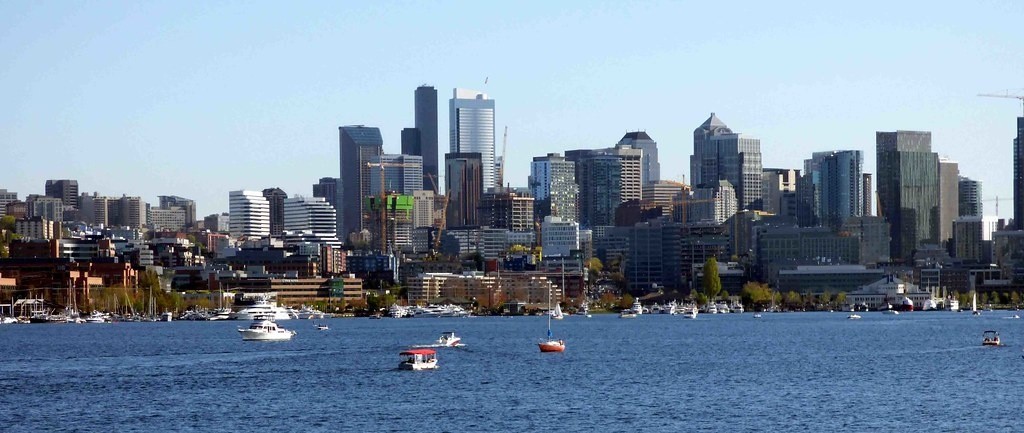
[
  {"x1": 982, "y1": 195, "x2": 1012, "y2": 222},
  {"x1": 424, "y1": 172, "x2": 454, "y2": 251},
  {"x1": 495, "y1": 125, "x2": 508, "y2": 195},
  {"x1": 360, "y1": 160, "x2": 422, "y2": 256},
  {"x1": 666, "y1": 175, "x2": 694, "y2": 225},
  {"x1": 874, "y1": 189, "x2": 883, "y2": 222},
  {"x1": 977, "y1": 90, "x2": 1024, "y2": 117},
  {"x1": 637, "y1": 192, "x2": 725, "y2": 223}
]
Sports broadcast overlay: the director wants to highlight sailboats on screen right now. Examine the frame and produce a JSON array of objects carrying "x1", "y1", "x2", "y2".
[
  {"x1": 971, "y1": 293, "x2": 981, "y2": 316},
  {"x1": 535, "y1": 280, "x2": 566, "y2": 352}
]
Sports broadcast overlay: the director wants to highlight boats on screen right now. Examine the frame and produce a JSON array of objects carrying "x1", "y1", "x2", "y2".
[
  {"x1": 982, "y1": 329, "x2": 1001, "y2": 345},
  {"x1": 368, "y1": 314, "x2": 382, "y2": 320},
  {"x1": 846, "y1": 314, "x2": 861, "y2": 319},
  {"x1": 318, "y1": 323, "x2": 328, "y2": 330},
  {"x1": 617, "y1": 296, "x2": 746, "y2": 320},
  {"x1": 387, "y1": 301, "x2": 473, "y2": 318},
  {"x1": 550, "y1": 302, "x2": 565, "y2": 320},
  {"x1": 237, "y1": 314, "x2": 297, "y2": 341},
  {"x1": 0, "y1": 285, "x2": 334, "y2": 323},
  {"x1": 882, "y1": 309, "x2": 900, "y2": 315},
  {"x1": 431, "y1": 331, "x2": 468, "y2": 348},
  {"x1": 398, "y1": 348, "x2": 441, "y2": 371}
]
